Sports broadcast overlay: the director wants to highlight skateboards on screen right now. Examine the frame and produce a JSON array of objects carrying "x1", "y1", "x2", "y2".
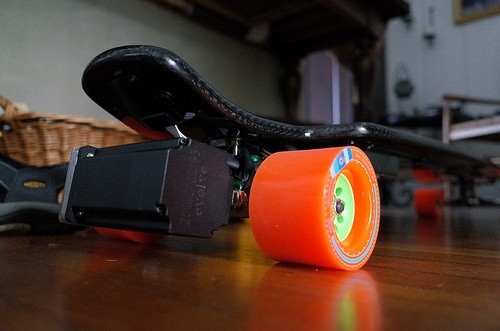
[{"x1": 58, "y1": 44, "x2": 500, "y2": 272}]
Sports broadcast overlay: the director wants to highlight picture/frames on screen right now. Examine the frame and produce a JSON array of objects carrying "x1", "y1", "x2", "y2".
[{"x1": 454, "y1": 0, "x2": 500, "y2": 25}]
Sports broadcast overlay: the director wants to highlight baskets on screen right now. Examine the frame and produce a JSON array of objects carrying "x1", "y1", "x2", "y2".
[{"x1": 1, "y1": 96, "x2": 148, "y2": 167}]
